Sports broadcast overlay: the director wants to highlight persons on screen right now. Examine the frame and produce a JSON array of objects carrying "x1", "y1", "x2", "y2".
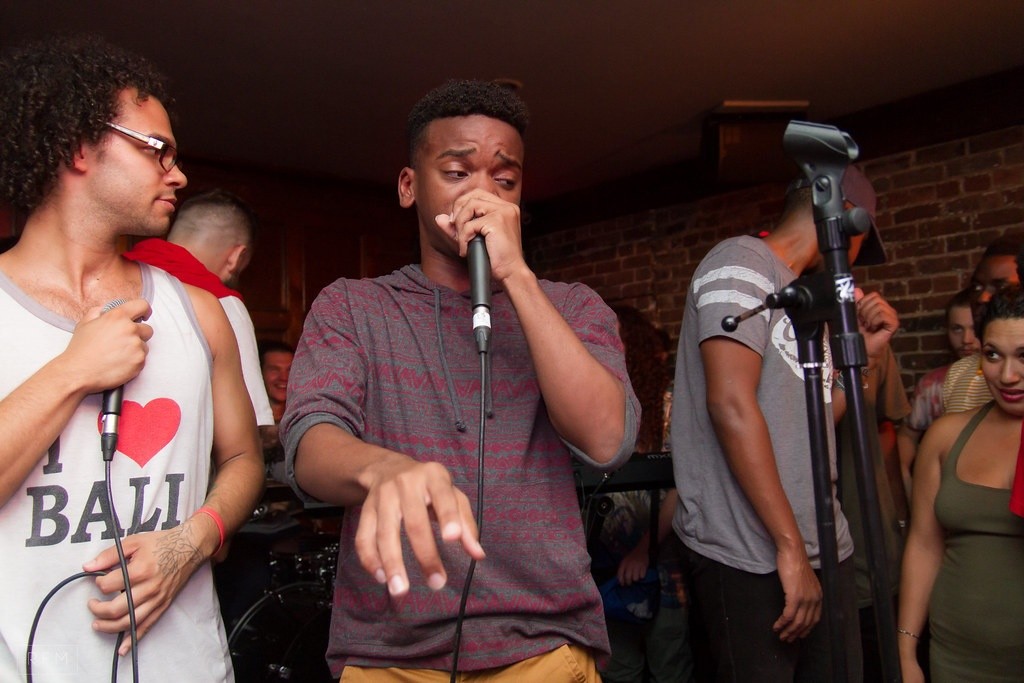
[
  {"x1": 672, "y1": 162, "x2": 897, "y2": 683},
  {"x1": 617, "y1": 285, "x2": 913, "y2": 683},
  {"x1": 116, "y1": 188, "x2": 277, "y2": 449},
  {"x1": 0, "y1": 38, "x2": 269, "y2": 683},
  {"x1": 278, "y1": 78, "x2": 642, "y2": 683},
  {"x1": 895, "y1": 287, "x2": 984, "y2": 506},
  {"x1": 897, "y1": 281, "x2": 1024, "y2": 683},
  {"x1": 234, "y1": 339, "x2": 295, "y2": 637},
  {"x1": 939, "y1": 236, "x2": 1023, "y2": 416},
  {"x1": 577, "y1": 489, "x2": 696, "y2": 683}
]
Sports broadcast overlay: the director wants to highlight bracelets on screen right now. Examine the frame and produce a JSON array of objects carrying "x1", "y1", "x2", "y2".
[
  {"x1": 191, "y1": 508, "x2": 226, "y2": 558},
  {"x1": 860, "y1": 366, "x2": 873, "y2": 379},
  {"x1": 897, "y1": 628, "x2": 920, "y2": 640},
  {"x1": 836, "y1": 370, "x2": 869, "y2": 393}
]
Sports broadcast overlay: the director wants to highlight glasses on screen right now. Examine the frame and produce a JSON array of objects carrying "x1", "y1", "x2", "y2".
[{"x1": 89, "y1": 108, "x2": 180, "y2": 175}]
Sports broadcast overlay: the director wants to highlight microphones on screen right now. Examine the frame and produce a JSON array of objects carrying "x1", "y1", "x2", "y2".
[
  {"x1": 467, "y1": 216, "x2": 493, "y2": 355},
  {"x1": 99, "y1": 298, "x2": 144, "y2": 460}
]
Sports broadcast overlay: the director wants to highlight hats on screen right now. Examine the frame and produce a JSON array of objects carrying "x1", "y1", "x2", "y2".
[{"x1": 783, "y1": 159, "x2": 888, "y2": 271}]
